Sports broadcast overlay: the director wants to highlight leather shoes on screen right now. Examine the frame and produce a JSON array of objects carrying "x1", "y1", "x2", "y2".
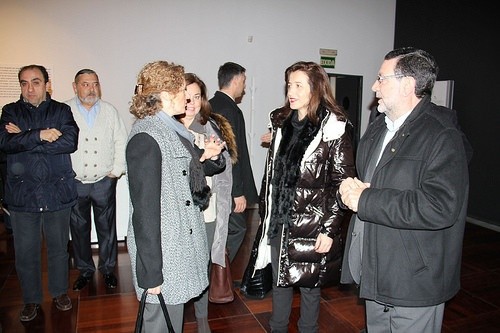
[
  {"x1": 102, "y1": 269, "x2": 119, "y2": 289},
  {"x1": 18, "y1": 301, "x2": 41, "y2": 322},
  {"x1": 51, "y1": 292, "x2": 73, "y2": 311},
  {"x1": 72, "y1": 268, "x2": 95, "y2": 291}
]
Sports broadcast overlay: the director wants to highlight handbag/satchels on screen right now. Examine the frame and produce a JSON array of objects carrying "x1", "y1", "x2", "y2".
[
  {"x1": 207, "y1": 247, "x2": 235, "y2": 304},
  {"x1": 239, "y1": 243, "x2": 273, "y2": 300}
]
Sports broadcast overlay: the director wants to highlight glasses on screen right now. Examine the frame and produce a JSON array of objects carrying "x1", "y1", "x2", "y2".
[{"x1": 376, "y1": 72, "x2": 416, "y2": 82}]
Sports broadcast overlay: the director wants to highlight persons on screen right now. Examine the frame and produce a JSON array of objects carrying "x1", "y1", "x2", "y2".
[
  {"x1": 59, "y1": 69, "x2": 126, "y2": 291},
  {"x1": 240, "y1": 61, "x2": 356, "y2": 333},
  {"x1": 0, "y1": 65, "x2": 79, "y2": 321},
  {"x1": 336, "y1": 45, "x2": 470, "y2": 333},
  {"x1": 124, "y1": 61, "x2": 227, "y2": 333},
  {"x1": 209, "y1": 62, "x2": 257, "y2": 266},
  {"x1": 261, "y1": 122, "x2": 273, "y2": 145},
  {"x1": 174, "y1": 73, "x2": 232, "y2": 333}
]
